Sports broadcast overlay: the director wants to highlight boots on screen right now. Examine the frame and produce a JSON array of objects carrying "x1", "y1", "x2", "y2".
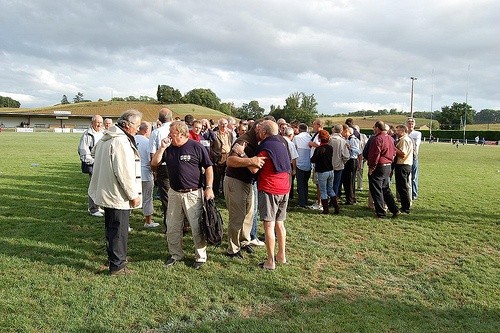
[
  {"x1": 332, "y1": 196, "x2": 340, "y2": 214},
  {"x1": 320, "y1": 199, "x2": 330, "y2": 214}
]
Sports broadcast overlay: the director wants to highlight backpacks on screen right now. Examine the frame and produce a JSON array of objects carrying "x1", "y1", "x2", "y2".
[{"x1": 199, "y1": 198, "x2": 223, "y2": 246}]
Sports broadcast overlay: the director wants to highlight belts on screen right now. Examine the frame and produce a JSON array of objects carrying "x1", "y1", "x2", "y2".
[{"x1": 174, "y1": 187, "x2": 200, "y2": 193}]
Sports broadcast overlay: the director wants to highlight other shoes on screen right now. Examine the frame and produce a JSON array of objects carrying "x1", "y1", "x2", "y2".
[
  {"x1": 227, "y1": 251, "x2": 244, "y2": 259},
  {"x1": 144, "y1": 221, "x2": 160, "y2": 227},
  {"x1": 90, "y1": 212, "x2": 103, "y2": 217},
  {"x1": 307, "y1": 202, "x2": 324, "y2": 211},
  {"x1": 166, "y1": 258, "x2": 176, "y2": 265},
  {"x1": 250, "y1": 237, "x2": 265, "y2": 246},
  {"x1": 241, "y1": 245, "x2": 255, "y2": 254},
  {"x1": 194, "y1": 262, "x2": 204, "y2": 269},
  {"x1": 125, "y1": 256, "x2": 131, "y2": 262},
  {"x1": 111, "y1": 266, "x2": 135, "y2": 276},
  {"x1": 88, "y1": 206, "x2": 105, "y2": 213}
]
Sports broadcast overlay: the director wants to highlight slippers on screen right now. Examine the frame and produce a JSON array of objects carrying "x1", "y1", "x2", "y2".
[{"x1": 259, "y1": 262, "x2": 273, "y2": 270}]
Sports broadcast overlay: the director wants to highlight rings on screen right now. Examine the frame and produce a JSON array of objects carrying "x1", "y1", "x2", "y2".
[{"x1": 236, "y1": 146, "x2": 239, "y2": 148}]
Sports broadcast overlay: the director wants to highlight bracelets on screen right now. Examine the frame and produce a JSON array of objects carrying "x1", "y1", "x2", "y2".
[{"x1": 206, "y1": 186, "x2": 212, "y2": 189}]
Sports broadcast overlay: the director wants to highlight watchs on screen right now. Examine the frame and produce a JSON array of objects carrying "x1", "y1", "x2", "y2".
[{"x1": 240, "y1": 152, "x2": 246, "y2": 158}]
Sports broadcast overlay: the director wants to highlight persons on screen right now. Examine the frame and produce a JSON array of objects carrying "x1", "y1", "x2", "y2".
[
  {"x1": 77, "y1": 108, "x2": 421, "y2": 232},
  {"x1": 223, "y1": 116, "x2": 292, "y2": 269},
  {"x1": 20, "y1": 121, "x2": 30, "y2": 128},
  {"x1": 456, "y1": 139, "x2": 460, "y2": 148},
  {"x1": 475, "y1": 136, "x2": 484, "y2": 145},
  {"x1": 88, "y1": 110, "x2": 143, "y2": 276},
  {"x1": 151, "y1": 122, "x2": 215, "y2": 270},
  {"x1": 429, "y1": 133, "x2": 434, "y2": 143}
]
